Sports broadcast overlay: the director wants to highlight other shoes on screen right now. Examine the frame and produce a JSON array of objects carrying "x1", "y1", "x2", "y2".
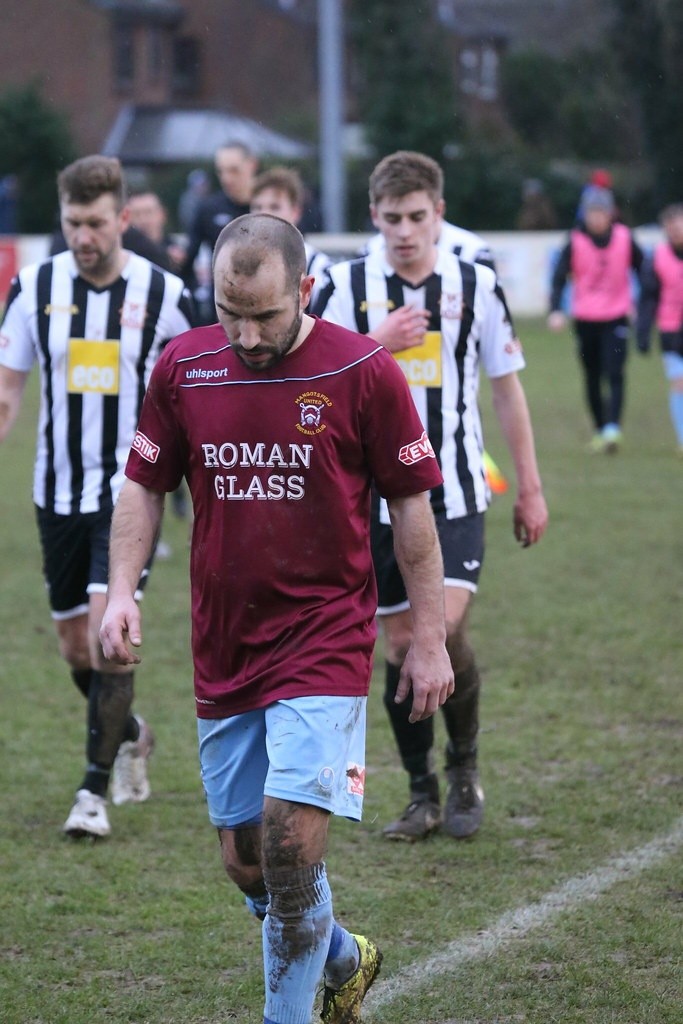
[{"x1": 591, "y1": 427, "x2": 621, "y2": 455}]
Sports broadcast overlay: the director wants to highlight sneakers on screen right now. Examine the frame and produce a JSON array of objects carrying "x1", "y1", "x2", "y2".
[
  {"x1": 62, "y1": 789, "x2": 111, "y2": 844},
  {"x1": 112, "y1": 714, "x2": 154, "y2": 805},
  {"x1": 445, "y1": 764, "x2": 485, "y2": 836},
  {"x1": 384, "y1": 792, "x2": 442, "y2": 843},
  {"x1": 316, "y1": 933, "x2": 383, "y2": 1024}
]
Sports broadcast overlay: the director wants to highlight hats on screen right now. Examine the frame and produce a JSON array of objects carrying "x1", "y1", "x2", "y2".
[{"x1": 580, "y1": 169, "x2": 616, "y2": 221}]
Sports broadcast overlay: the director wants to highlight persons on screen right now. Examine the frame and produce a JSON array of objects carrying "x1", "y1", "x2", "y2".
[
  {"x1": 0, "y1": 154, "x2": 195, "y2": 838},
  {"x1": 50, "y1": 183, "x2": 203, "y2": 326},
  {"x1": 177, "y1": 168, "x2": 210, "y2": 228},
  {"x1": 355, "y1": 216, "x2": 508, "y2": 494},
  {"x1": 636, "y1": 204, "x2": 683, "y2": 447},
  {"x1": 248, "y1": 167, "x2": 332, "y2": 314},
  {"x1": 98, "y1": 212, "x2": 455, "y2": 1023},
  {"x1": 181, "y1": 143, "x2": 258, "y2": 266},
  {"x1": 546, "y1": 170, "x2": 645, "y2": 452},
  {"x1": 310, "y1": 152, "x2": 548, "y2": 843}
]
